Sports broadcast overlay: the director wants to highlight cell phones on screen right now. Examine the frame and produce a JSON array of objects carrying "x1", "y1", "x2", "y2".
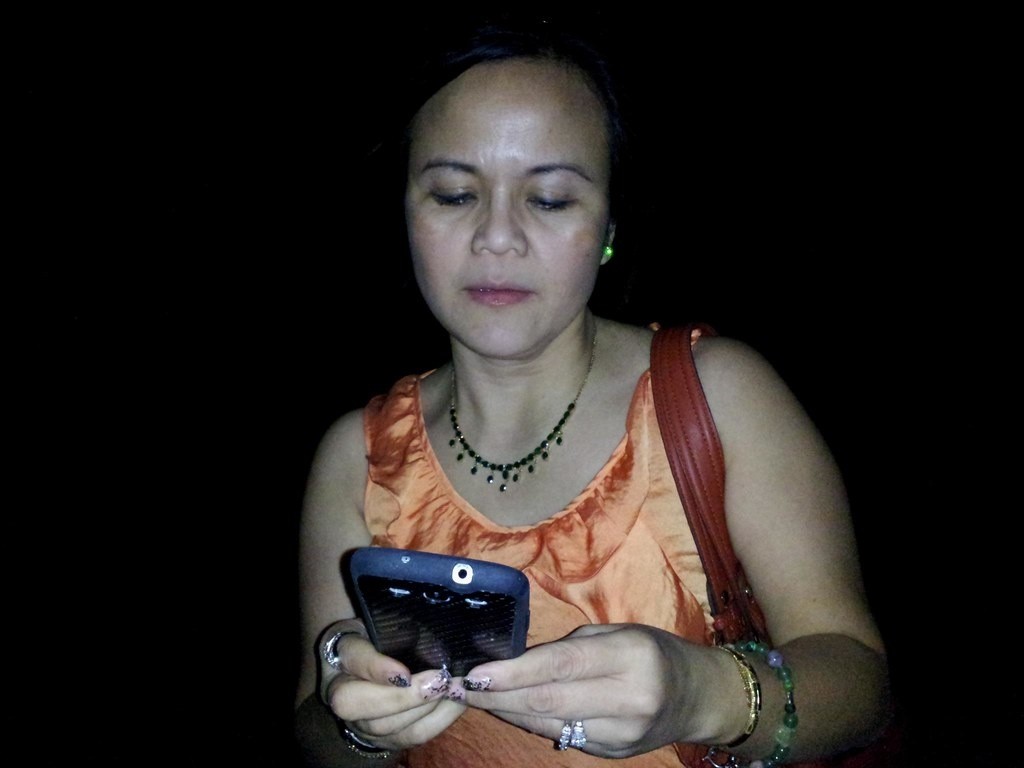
[{"x1": 350, "y1": 547, "x2": 530, "y2": 677}]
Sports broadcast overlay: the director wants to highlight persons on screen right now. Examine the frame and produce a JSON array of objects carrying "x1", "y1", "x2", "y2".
[{"x1": 286, "y1": 22, "x2": 908, "y2": 767}]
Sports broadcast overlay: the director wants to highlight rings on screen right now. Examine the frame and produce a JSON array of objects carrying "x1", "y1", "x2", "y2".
[
  {"x1": 322, "y1": 632, "x2": 363, "y2": 676},
  {"x1": 559, "y1": 718, "x2": 573, "y2": 750},
  {"x1": 568, "y1": 719, "x2": 587, "y2": 749}
]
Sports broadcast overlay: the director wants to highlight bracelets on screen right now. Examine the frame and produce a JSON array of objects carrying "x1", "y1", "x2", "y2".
[
  {"x1": 700, "y1": 636, "x2": 801, "y2": 768},
  {"x1": 340, "y1": 724, "x2": 395, "y2": 759}
]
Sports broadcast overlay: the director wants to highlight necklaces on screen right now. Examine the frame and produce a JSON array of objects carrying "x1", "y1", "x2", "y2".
[{"x1": 448, "y1": 314, "x2": 600, "y2": 493}]
